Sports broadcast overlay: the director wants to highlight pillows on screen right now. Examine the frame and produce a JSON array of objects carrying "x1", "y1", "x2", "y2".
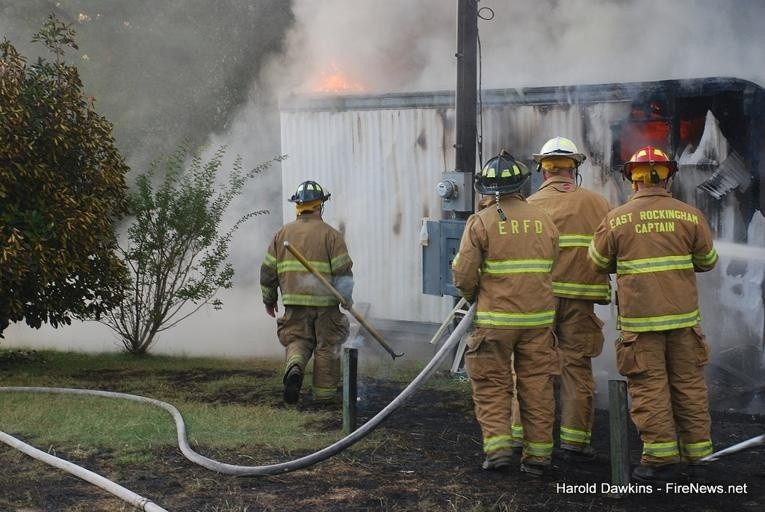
[
  {"x1": 532, "y1": 136, "x2": 586, "y2": 164},
  {"x1": 624, "y1": 145, "x2": 679, "y2": 181},
  {"x1": 288, "y1": 181, "x2": 330, "y2": 203},
  {"x1": 474, "y1": 152, "x2": 530, "y2": 195}
]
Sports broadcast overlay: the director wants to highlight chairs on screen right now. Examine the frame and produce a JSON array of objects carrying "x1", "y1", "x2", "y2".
[
  {"x1": 283, "y1": 365, "x2": 302, "y2": 404},
  {"x1": 632, "y1": 464, "x2": 709, "y2": 484},
  {"x1": 482, "y1": 437, "x2": 598, "y2": 476},
  {"x1": 314, "y1": 398, "x2": 341, "y2": 409}
]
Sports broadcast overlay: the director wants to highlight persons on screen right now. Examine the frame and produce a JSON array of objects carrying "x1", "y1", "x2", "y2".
[
  {"x1": 259, "y1": 181, "x2": 355, "y2": 410},
  {"x1": 585, "y1": 146, "x2": 719, "y2": 488},
  {"x1": 512, "y1": 133, "x2": 613, "y2": 474},
  {"x1": 451, "y1": 146, "x2": 561, "y2": 479}
]
